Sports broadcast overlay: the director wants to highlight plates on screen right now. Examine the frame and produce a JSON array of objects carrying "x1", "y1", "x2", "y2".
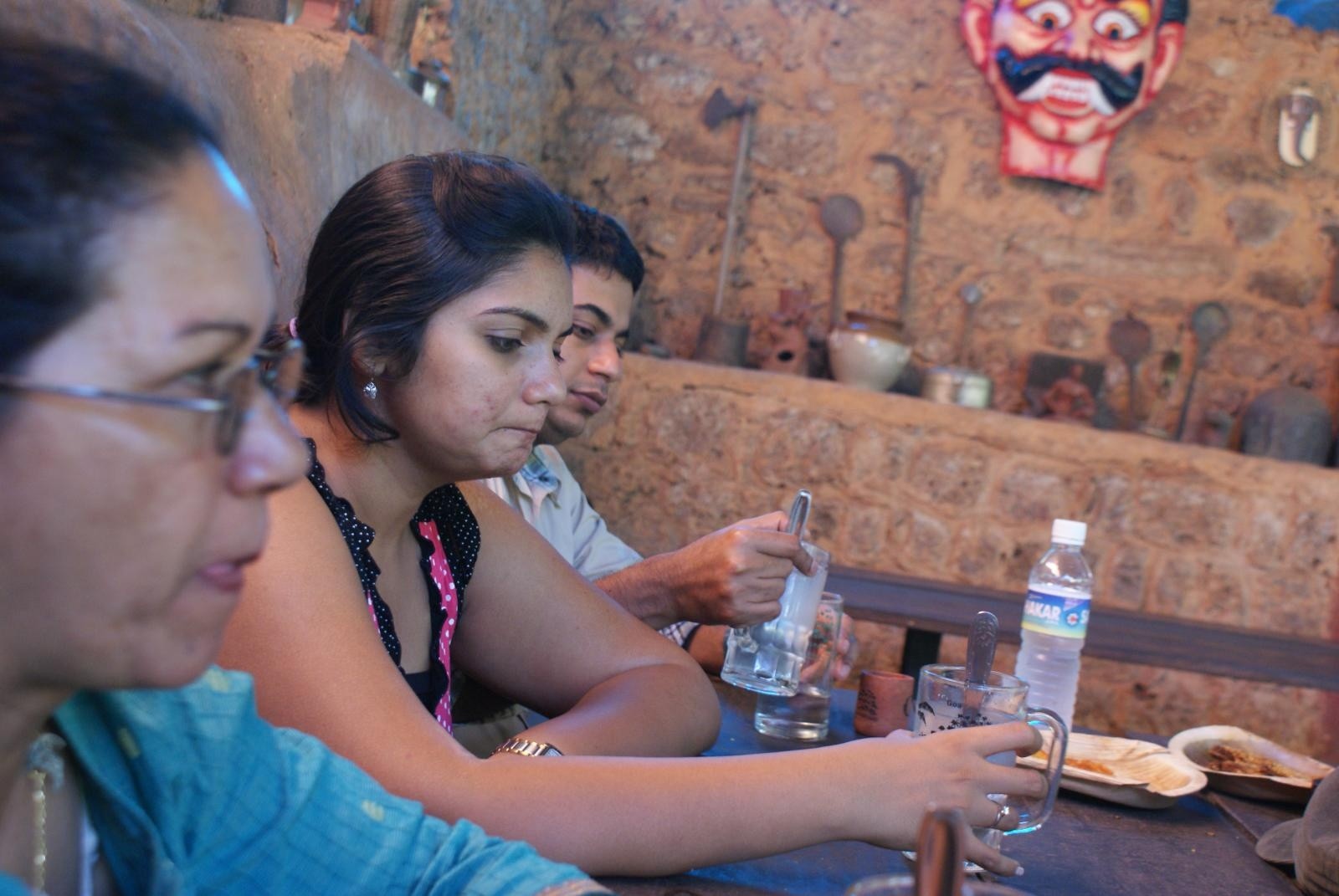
[
  {"x1": 1167, "y1": 724, "x2": 1336, "y2": 802},
  {"x1": 1015, "y1": 727, "x2": 1208, "y2": 809}
]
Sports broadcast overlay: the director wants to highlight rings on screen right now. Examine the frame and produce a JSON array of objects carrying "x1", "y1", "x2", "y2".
[{"x1": 990, "y1": 798, "x2": 1010, "y2": 827}]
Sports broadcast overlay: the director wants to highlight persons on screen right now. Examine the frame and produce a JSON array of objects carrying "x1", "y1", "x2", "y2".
[
  {"x1": 185, "y1": 141, "x2": 1050, "y2": 879},
  {"x1": 471, "y1": 186, "x2": 860, "y2": 694},
  {"x1": 0, "y1": 39, "x2": 622, "y2": 896}
]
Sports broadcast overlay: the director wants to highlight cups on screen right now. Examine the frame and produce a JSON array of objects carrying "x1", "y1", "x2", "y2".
[
  {"x1": 902, "y1": 664, "x2": 1067, "y2": 871},
  {"x1": 920, "y1": 369, "x2": 990, "y2": 407},
  {"x1": 720, "y1": 540, "x2": 830, "y2": 696},
  {"x1": 855, "y1": 669, "x2": 914, "y2": 736},
  {"x1": 753, "y1": 591, "x2": 843, "y2": 740},
  {"x1": 407, "y1": 59, "x2": 450, "y2": 109}
]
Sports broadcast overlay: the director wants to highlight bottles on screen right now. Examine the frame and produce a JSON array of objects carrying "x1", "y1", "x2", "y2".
[{"x1": 1013, "y1": 518, "x2": 1092, "y2": 732}]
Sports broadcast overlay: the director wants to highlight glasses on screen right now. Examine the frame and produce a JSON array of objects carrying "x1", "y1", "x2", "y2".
[{"x1": 1, "y1": 364, "x2": 254, "y2": 456}]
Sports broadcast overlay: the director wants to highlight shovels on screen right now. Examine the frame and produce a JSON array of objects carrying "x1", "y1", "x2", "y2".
[
  {"x1": 820, "y1": 194, "x2": 866, "y2": 336},
  {"x1": 1109, "y1": 319, "x2": 1153, "y2": 410}
]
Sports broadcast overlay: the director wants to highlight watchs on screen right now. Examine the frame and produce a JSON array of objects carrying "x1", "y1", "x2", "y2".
[{"x1": 491, "y1": 738, "x2": 564, "y2": 757}]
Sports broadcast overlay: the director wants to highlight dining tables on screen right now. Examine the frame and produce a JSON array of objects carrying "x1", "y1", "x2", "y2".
[{"x1": 583, "y1": 666, "x2": 1298, "y2": 896}]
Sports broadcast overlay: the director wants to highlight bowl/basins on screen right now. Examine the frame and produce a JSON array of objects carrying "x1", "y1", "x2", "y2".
[{"x1": 824, "y1": 321, "x2": 915, "y2": 391}]
